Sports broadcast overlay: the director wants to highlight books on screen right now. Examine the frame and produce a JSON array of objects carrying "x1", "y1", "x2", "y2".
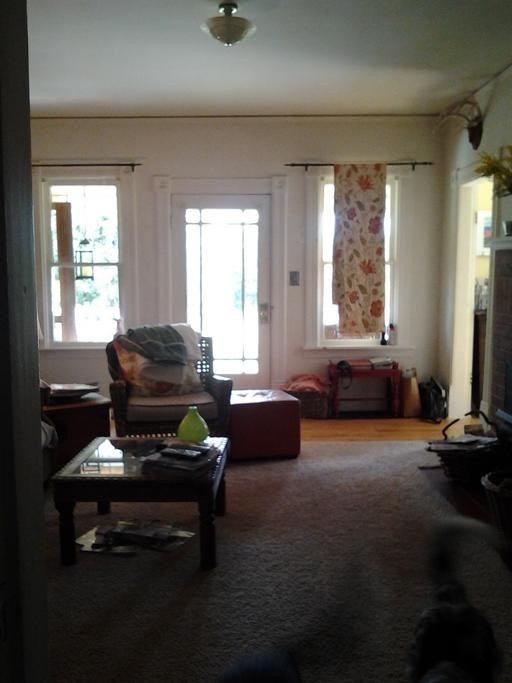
[
  {"x1": 348, "y1": 355, "x2": 394, "y2": 371},
  {"x1": 140, "y1": 437, "x2": 222, "y2": 478}
]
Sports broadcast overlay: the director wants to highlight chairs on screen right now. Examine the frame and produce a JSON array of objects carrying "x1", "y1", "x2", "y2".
[{"x1": 106, "y1": 324, "x2": 234, "y2": 438}]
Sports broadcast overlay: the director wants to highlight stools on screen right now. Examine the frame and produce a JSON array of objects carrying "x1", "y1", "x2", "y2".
[{"x1": 226, "y1": 388, "x2": 303, "y2": 461}]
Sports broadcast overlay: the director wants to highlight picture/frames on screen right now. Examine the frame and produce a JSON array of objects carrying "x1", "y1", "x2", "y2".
[{"x1": 475, "y1": 209, "x2": 493, "y2": 256}]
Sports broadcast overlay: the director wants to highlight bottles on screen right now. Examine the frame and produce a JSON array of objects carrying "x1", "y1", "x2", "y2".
[{"x1": 176, "y1": 406, "x2": 209, "y2": 443}]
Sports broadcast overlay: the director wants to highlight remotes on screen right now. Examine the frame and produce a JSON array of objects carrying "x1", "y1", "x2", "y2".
[{"x1": 160, "y1": 444, "x2": 210, "y2": 459}]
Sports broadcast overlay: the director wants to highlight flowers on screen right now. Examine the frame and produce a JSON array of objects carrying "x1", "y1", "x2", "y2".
[{"x1": 475, "y1": 149, "x2": 512, "y2": 199}]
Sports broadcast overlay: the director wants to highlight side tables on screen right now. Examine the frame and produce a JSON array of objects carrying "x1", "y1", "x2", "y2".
[{"x1": 43, "y1": 393, "x2": 113, "y2": 472}]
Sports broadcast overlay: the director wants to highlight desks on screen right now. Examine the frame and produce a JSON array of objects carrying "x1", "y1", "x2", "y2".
[{"x1": 328, "y1": 364, "x2": 400, "y2": 419}]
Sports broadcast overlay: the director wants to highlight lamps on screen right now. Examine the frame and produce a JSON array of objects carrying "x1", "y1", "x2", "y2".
[
  {"x1": 199, "y1": 3, "x2": 257, "y2": 47},
  {"x1": 74, "y1": 239, "x2": 94, "y2": 280}
]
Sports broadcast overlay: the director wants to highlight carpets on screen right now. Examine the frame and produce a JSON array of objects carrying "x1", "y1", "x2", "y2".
[{"x1": 40, "y1": 440, "x2": 512, "y2": 683}]
